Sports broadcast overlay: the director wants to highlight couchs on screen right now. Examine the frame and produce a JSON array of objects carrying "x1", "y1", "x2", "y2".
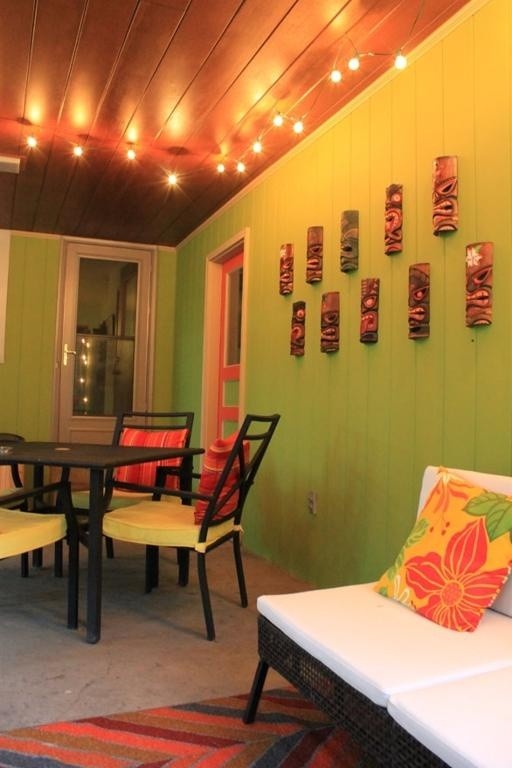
[{"x1": 245, "y1": 458, "x2": 512, "y2": 765}]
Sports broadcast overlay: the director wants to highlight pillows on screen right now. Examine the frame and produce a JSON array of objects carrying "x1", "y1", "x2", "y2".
[
  {"x1": 368, "y1": 471, "x2": 512, "y2": 633},
  {"x1": 115, "y1": 425, "x2": 189, "y2": 492},
  {"x1": 195, "y1": 432, "x2": 250, "y2": 527}
]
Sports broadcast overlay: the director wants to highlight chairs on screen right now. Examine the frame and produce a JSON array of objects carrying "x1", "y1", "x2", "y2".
[
  {"x1": 102, "y1": 414, "x2": 282, "y2": 639},
  {"x1": 0, "y1": 504, "x2": 81, "y2": 630},
  {"x1": 66, "y1": 408, "x2": 195, "y2": 566}
]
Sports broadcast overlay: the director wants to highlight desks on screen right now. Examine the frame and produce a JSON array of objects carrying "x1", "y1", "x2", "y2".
[{"x1": 5, "y1": 440, "x2": 204, "y2": 644}]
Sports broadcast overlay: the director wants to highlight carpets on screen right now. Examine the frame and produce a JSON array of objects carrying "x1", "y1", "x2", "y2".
[{"x1": 1, "y1": 679, "x2": 387, "y2": 762}]
